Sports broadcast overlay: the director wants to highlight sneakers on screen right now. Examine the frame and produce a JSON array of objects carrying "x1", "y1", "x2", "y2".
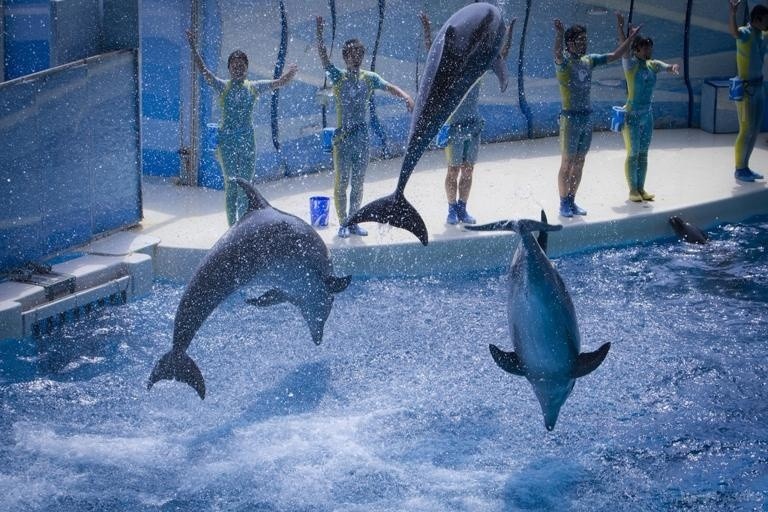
[
  {"x1": 637, "y1": 189, "x2": 655, "y2": 200},
  {"x1": 749, "y1": 168, "x2": 764, "y2": 180},
  {"x1": 337, "y1": 223, "x2": 350, "y2": 238},
  {"x1": 348, "y1": 222, "x2": 370, "y2": 236},
  {"x1": 628, "y1": 190, "x2": 643, "y2": 202},
  {"x1": 734, "y1": 168, "x2": 755, "y2": 183}
]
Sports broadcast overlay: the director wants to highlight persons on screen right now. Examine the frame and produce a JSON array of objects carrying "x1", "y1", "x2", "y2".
[
  {"x1": 553, "y1": 17, "x2": 643, "y2": 220},
  {"x1": 315, "y1": 15, "x2": 414, "y2": 237},
  {"x1": 418, "y1": 11, "x2": 517, "y2": 226},
  {"x1": 185, "y1": 29, "x2": 298, "y2": 224},
  {"x1": 614, "y1": 10, "x2": 680, "y2": 201},
  {"x1": 726, "y1": 0, "x2": 768, "y2": 182}
]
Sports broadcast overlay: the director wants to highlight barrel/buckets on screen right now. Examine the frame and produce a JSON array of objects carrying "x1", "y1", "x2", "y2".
[
  {"x1": 610, "y1": 105, "x2": 626, "y2": 133},
  {"x1": 206, "y1": 122, "x2": 219, "y2": 152},
  {"x1": 728, "y1": 77, "x2": 745, "y2": 102},
  {"x1": 436, "y1": 124, "x2": 451, "y2": 148},
  {"x1": 310, "y1": 195, "x2": 330, "y2": 227},
  {"x1": 321, "y1": 127, "x2": 336, "y2": 155}
]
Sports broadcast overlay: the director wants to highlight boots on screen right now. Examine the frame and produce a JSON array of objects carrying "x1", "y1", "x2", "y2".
[
  {"x1": 568, "y1": 193, "x2": 587, "y2": 216},
  {"x1": 558, "y1": 196, "x2": 573, "y2": 217},
  {"x1": 446, "y1": 202, "x2": 458, "y2": 225},
  {"x1": 458, "y1": 199, "x2": 478, "y2": 226}
]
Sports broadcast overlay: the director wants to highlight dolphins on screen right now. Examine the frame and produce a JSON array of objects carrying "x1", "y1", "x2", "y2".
[
  {"x1": 338, "y1": 2, "x2": 510, "y2": 247},
  {"x1": 463, "y1": 208, "x2": 612, "y2": 432},
  {"x1": 146, "y1": 175, "x2": 353, "y2": 401},
  {"x1": 669, "y1": 215, "x2": 707, "y2": 245}
]
